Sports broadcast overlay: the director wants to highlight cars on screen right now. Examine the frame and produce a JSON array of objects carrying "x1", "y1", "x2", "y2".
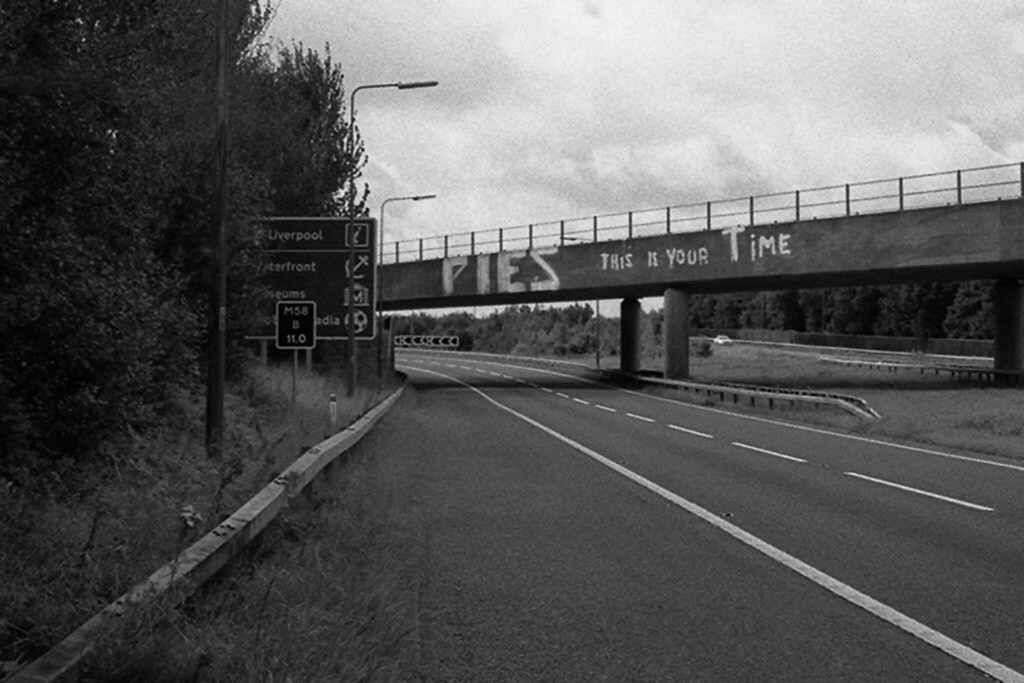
[{"x1": 714, "y1": 335, "x2": 732, "y2": 346}]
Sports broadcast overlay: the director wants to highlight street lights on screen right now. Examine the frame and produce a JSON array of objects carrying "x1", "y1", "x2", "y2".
[
  {"x1": 346, "y1": 80, "x2": 440, "y2": 399},
  {"x1": 376, "y1": 193, "x2": 437, "y2": 380}
]
